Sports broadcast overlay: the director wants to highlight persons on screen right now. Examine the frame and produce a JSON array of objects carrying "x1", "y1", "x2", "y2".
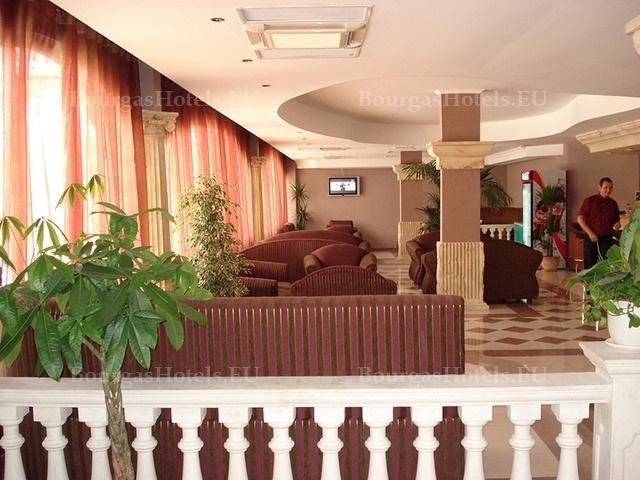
[{"x1": 575, "y1": 175, "x2": 621, "y2": 270}]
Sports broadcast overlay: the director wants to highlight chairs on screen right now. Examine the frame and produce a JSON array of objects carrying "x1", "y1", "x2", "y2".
[
  {"x1": 234, "y1": 220, "x2": 397, "y2": 296},
  {"x1": 567, "y1": 222, "x2": 620, "y2": 272}
]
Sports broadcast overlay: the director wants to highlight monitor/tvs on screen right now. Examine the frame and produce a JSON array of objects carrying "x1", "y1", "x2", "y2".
[{"x1": 328, "y1": 175, "x2": 361, "y2": 196}]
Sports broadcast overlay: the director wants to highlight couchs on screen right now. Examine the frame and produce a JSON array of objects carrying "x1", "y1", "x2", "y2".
[{"x1": 406, "y1": 230, "x2": 544, "y2": 305}]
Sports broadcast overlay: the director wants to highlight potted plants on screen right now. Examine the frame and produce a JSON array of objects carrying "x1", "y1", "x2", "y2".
[
  {"x1": 535, "y1": 184, "x2": 562, "y2": 272},
  {"x1": 564, "y1": 206, "x2": 640, "y2": 347}
]
